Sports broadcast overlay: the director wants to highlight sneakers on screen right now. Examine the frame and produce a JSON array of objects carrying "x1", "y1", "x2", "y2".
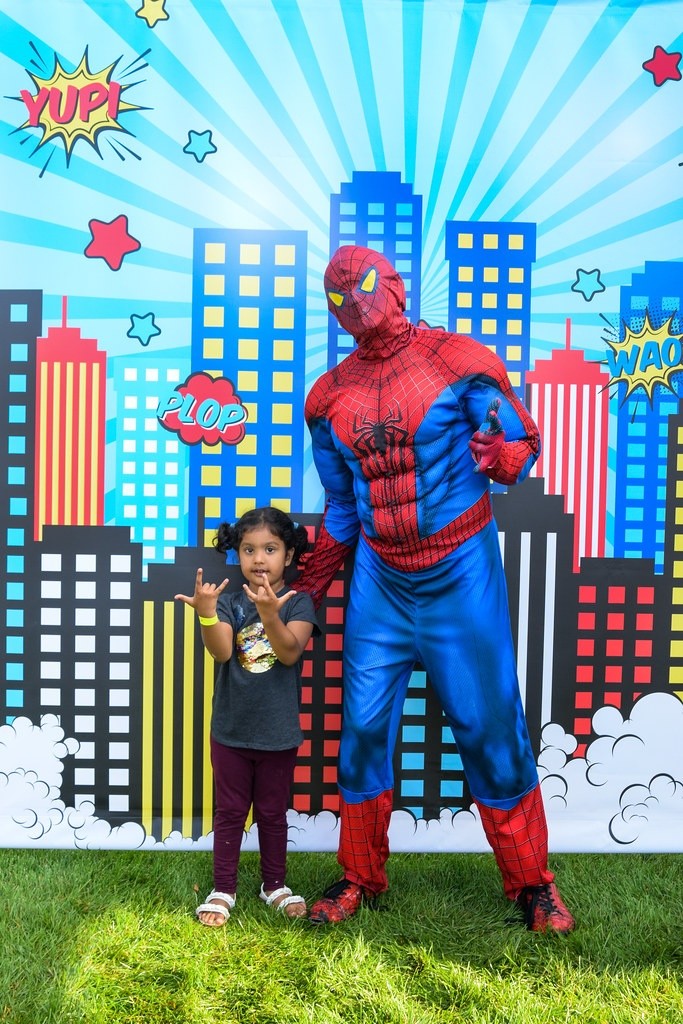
[
  {"x1": 518, "y1": 881, "x2": 578, "y2": 933},
  {"x1": 311, "y1": 874, "x2": 375, "y2": 925}
]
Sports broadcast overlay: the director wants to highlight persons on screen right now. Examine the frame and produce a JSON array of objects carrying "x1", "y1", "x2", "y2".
[
  {"x1": 172, "y1": 507, "x2": 322, "y2": 927},
  {"x1": 291, "y1": 244, "x2": 575, "y2": 938}
]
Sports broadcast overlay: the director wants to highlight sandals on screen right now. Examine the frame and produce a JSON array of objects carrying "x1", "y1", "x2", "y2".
[
  {"x1": 198, "y1": 888, "x2": 237, "y2": 926},
  {"x1": 258, "y1": 881, "x2": 307, "y2": 919}
]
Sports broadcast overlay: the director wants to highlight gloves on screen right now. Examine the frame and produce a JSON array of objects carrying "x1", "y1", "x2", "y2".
[{"x1": 468, "y1": 398, "x2": 506, "y2": 474}]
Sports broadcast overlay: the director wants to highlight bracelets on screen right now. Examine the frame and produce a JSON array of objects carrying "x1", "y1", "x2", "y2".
[{"x1": 198, "y1": 615, "x2": 218, "y2": 626}]
